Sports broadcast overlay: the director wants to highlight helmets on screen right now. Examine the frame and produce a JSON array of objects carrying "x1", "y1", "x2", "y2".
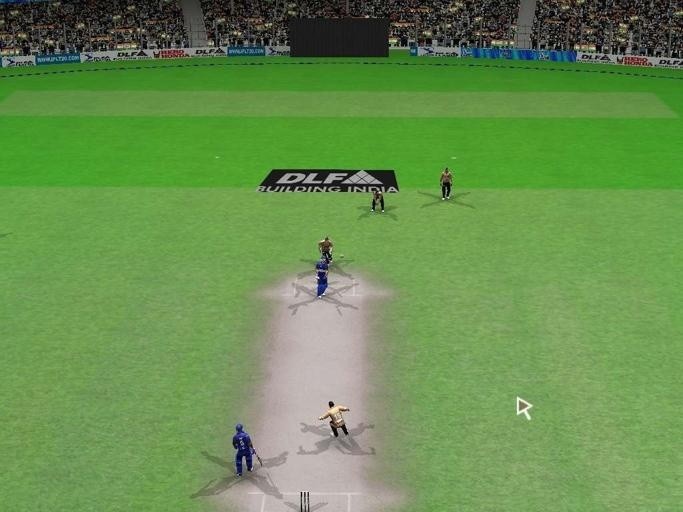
[{"x1": 236, "y1": 424, "x2": 243, "y2": 430}]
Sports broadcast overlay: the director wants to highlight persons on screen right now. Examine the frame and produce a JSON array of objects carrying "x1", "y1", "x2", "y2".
[
  {"x1": 317, "y1": 237, "x2": 334, "y2": 265},
  {"x1": 1, "y1": 1, "x2": 682, "y2": 68},
  {"x1": 316, "y1": 400, "x2": 351, "y2": 438},
  {"x1": 313, "y1": 262, "x2": 329, "y2": 299},
  {"x1": 232, "y1": 421, "x2": 254, "y2": 478},
  {"x1": 369, "y1": 186, "x2": 386, "y2": 214},
  {"x1": 437, "y1": 165, "x2": 454, "y2": 203}
]
